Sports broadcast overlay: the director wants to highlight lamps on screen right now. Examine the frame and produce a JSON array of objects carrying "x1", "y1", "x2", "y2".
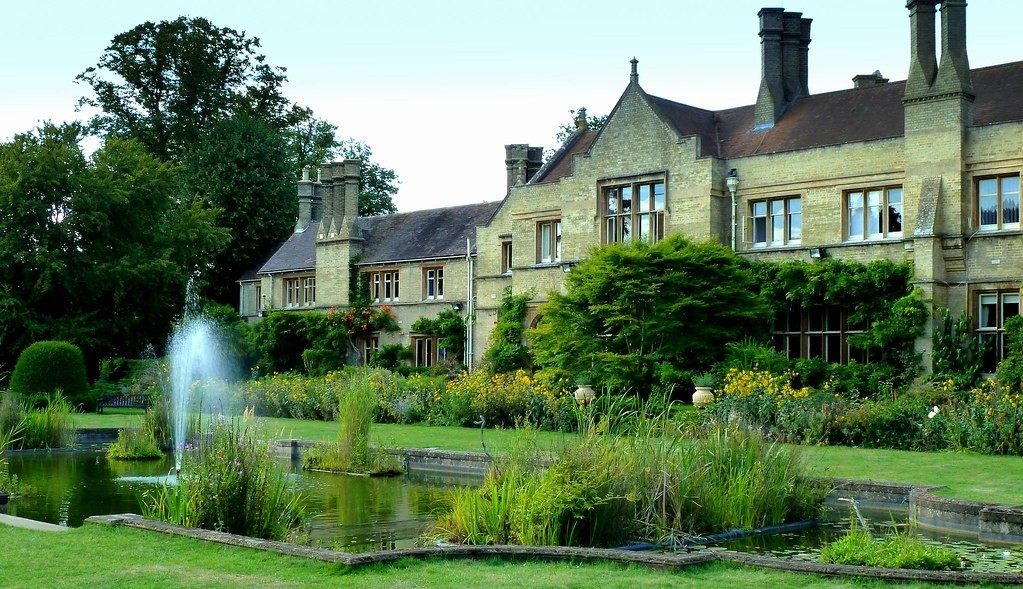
[
  {"x1": 450, "y1": 302, "x2": 463, "y2": 311},
  {"x1": 562, "y1": 263, "x2": 577, "y2": 274},
  {"x1": 810, "y1": 247, "x2": 827, "y2": 261},
  {"x1": 257, "y1": 311, "x2": 267, "y2": 318}
]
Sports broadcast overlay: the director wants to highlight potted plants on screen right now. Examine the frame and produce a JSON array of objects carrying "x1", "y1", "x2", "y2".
[
  {"x1": 690, "y1": 371, "x2": 714, "y2": 409},
  {"x1": 574, "y1": 373, "x2": 595, "y2": 405}
]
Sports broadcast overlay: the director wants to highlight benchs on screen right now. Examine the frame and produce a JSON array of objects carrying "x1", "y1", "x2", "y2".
[{"x1": 96, "y1": 393, "x2": 154, "y2": 416}]
